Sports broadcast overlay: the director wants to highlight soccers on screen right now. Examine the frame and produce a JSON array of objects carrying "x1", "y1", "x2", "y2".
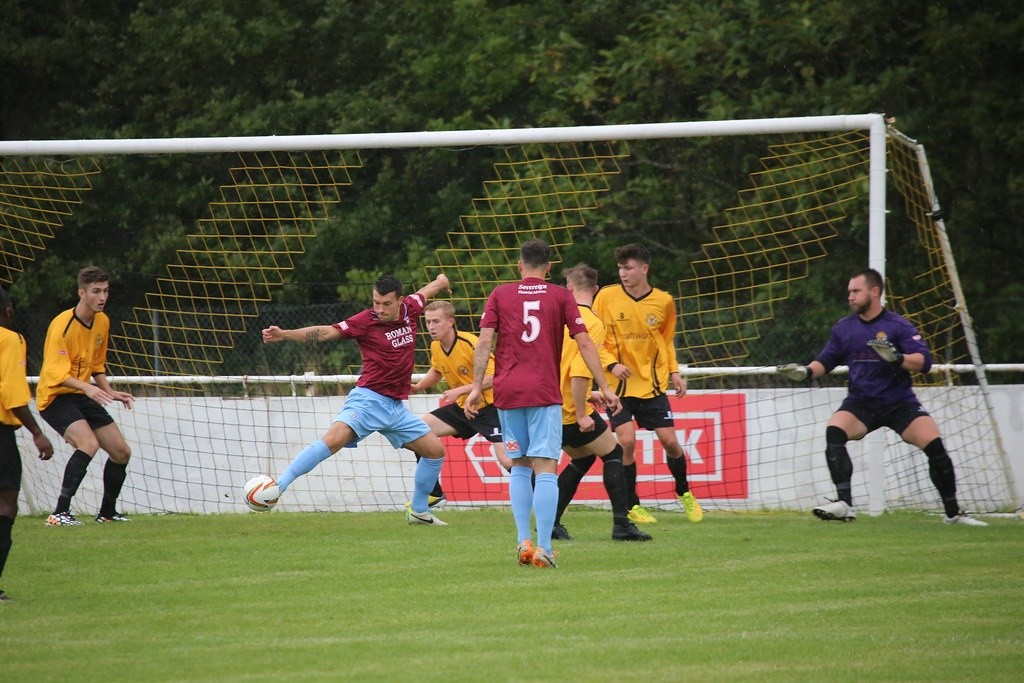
[{"x1": 243, "y1": 474, "x2": 281, "y2": 512}]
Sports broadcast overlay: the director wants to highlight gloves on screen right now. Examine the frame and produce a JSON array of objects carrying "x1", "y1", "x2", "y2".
[
  {"x1": 776, "y1": 364, "x2": 812, "y2": 385},
  {"x1": 866, "y1": 340, "x2": 904, "y2": 366}
]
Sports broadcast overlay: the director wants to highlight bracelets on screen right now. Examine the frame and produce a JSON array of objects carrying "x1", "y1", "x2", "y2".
[
  {"x1": 607, "y1": 363, "x2": 616, "y2": 372},
  {"x1": 35, "y1": 431, "x2": 42, "y2": 437}
]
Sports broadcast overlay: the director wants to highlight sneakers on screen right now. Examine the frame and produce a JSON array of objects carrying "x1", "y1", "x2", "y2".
[
  {"x1": 627, "y1": 505, "x2": 657, "y2": 523},
  {"x1": 943, "y1": 512, "x2": 990, "y2": 527},
  {"x1": 612, "y1": 521, "x2": 655, "y2": 541},
  {"x1": 515, "y1": 540, "x2": 533, "y2": 564},
  {"x1": 408, "y1": 509, "x2": 447, "y2": 528},
  {"x1": 44, "y1": 511, "x2": 84, "y2": 527},
  {"x1": 93, "y1": 512, "x2": 132, "y2": 523},
  {"x1": 675, "y1": 488, "x2": 703, "y2": 523},
  {"x1": 404, "y1": 491, "x2": 446, "y2": 509},
  {"x1": 535, "y1": 524, "x2": 571, "y2": 541},
  {"x1": 531, "y1": 546, "x2": 558, "y2": 569},
  {"x1": 812, "y1": 497, "x2": 856, "y2": 522}
]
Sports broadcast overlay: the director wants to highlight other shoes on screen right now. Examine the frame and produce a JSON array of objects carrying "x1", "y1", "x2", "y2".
[{"x1": 0, "y1": 590, "x2": 12, "y2": 603}]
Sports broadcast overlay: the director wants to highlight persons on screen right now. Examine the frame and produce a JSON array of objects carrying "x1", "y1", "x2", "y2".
[
  {"x1": 592, "y1": 242, "x2": 703, "y2": 524},
  {"x1": 405, "y1": 301, "x2": 537, "y2": 508},
  {"x1": 534, "y1": 261, "x2": 652, "y2": 540},
  {"x1": 36, "y1": 265, "x2": 136, "y2": 526},
  {"x1": 777, "y1": 268, "x2": 989, "y2": 526},
  {"x1": 463, "y1": 240, "x2": 623, "y2": 568},
  {"x1": 0, "y1": 286, "x2": 54, "y2": 603},
  {"x1": 262, "y1": 273, "x2": 453, "y2": 527}
]
